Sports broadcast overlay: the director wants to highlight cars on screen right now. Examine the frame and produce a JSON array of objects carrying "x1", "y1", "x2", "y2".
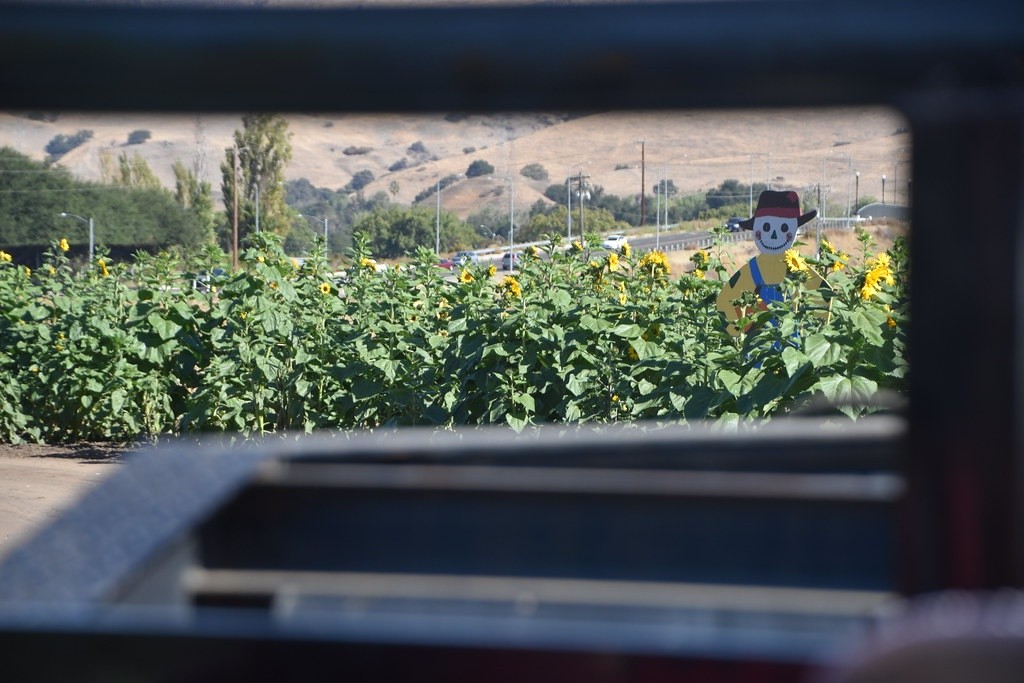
[
  {"x1": 724, "y1": 216, "x2": 747, "y2": 233},
  {"x1": 430, "y1": 258, "x2": 455, "y2": 271},
  {"x1": 603, "y1": 235, "x2": 626, "y2": 251},
  {"x1": 190, "y1": 267, "x2": 224, "y2": 288},
  {"x1": 454, "y1": 251, "x2": 483, "y2": 267},
  {"x1": 502, "y1": 250, "x2": 525, "y2": 270}
]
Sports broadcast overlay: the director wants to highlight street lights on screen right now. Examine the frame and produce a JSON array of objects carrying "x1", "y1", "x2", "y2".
[
  {"x1": 252, "y1": 183, "x2": 260, "y2": 235},
  {"x1": 854, "y1": 172, "x2": 860, "y2": 213},
  {"x1": 822, "y1": 152, "x2": 843, "y2": 222},
  {"x1": 882, "y1": 175, "x2": 887, "y2": 204},
  {"x1": 907, "y1": 178, "x2": 914, "y2": 208},
  {"x1": 635, "y1": 163, "x2": 661, "y2": 252},
  {"x1": 436, "y1": 173, "x2": 466, "y2": 255},
  {"x1": 750, "y1": 152, "x2": 773, "y2": 220},
  {"x1": 746, "y1": 154, "x2": 769, "y2": 191},
  {"x1": 298, "y1": 214, "x2": 328, "y2": 266},
  {"x1": 60, "y1": 211, "x2": 94, "y2": 270},
  {"x1": 664, "y1": 153, "x2": 688, "y2": 232},
  {"x1": 487, "y1": 176, "x2": 514, "y2": 274},
  {"x1": 893, "y1": 160, "x2": 912, "y2": 205},
  {"x1": 568, "y1": 161, "x2": 593, "y2": 246}
]
